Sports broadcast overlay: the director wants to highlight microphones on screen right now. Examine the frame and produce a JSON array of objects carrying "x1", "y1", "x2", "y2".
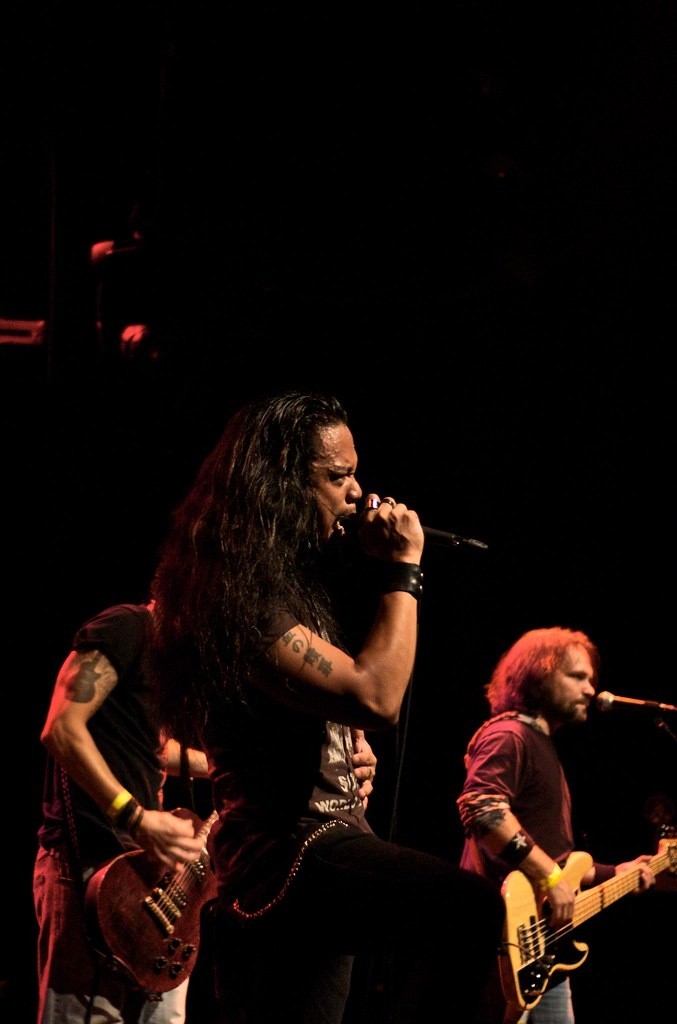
[
  {"x1": 597, "y1": 692, "x2": 677, "y2": 714},
  {"x1": 350, "y1": 510, "x2": 489, "y2": 554}
]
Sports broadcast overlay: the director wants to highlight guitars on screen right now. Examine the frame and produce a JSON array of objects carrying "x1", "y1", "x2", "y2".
[
  {"x1": 84, "y1": 792, "x2": 237, "y2": 997},
  {"x1": 497, "y1": 823, "x2": 677, "y2": 1012}
]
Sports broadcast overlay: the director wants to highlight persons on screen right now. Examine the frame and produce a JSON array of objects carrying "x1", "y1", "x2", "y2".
[
  {"x1": 151, "y1": 392, "x2": 505, "y2": 1024},
  {"x1": 31, "y1": 542, "x2": 219, "y2": 1002},
  {"x1": 456, "y1": 627, "x2": 656, "y2": 1024}
]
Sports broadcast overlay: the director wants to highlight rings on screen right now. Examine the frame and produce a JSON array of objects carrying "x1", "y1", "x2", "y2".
[
  {"x1": 382, "y1": 499, "x2": 394, "y2": 508},
  {"x1": 369, "y1": 767, "x2": 375, "y2": 777},
  {"x1": 364, "y1": 499, "x2": 379, "y2": 510}
]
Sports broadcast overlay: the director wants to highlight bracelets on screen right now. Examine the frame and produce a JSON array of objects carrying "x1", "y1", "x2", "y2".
[
  {"x1": 106, "y1": 789, "x2": 132, "y2": 820},
  {"x1": 181, "y1": 745, "x2": 190, "y2": 778},
  {"x1": 539, "y1": 863, "x2": 564, "y2": 892},
  {"x1": 114, "y1": 799, "x2": 144, "y2": 839},
  {"x1": 593, "y1": 863, "x2": 615, "y2": 886},
  {"x1": 378, "y1": 562, "x2": 423, "y2": 599},
  {"x1": 498, "y1": 828, "x2": 536, "y2": 865}
]
